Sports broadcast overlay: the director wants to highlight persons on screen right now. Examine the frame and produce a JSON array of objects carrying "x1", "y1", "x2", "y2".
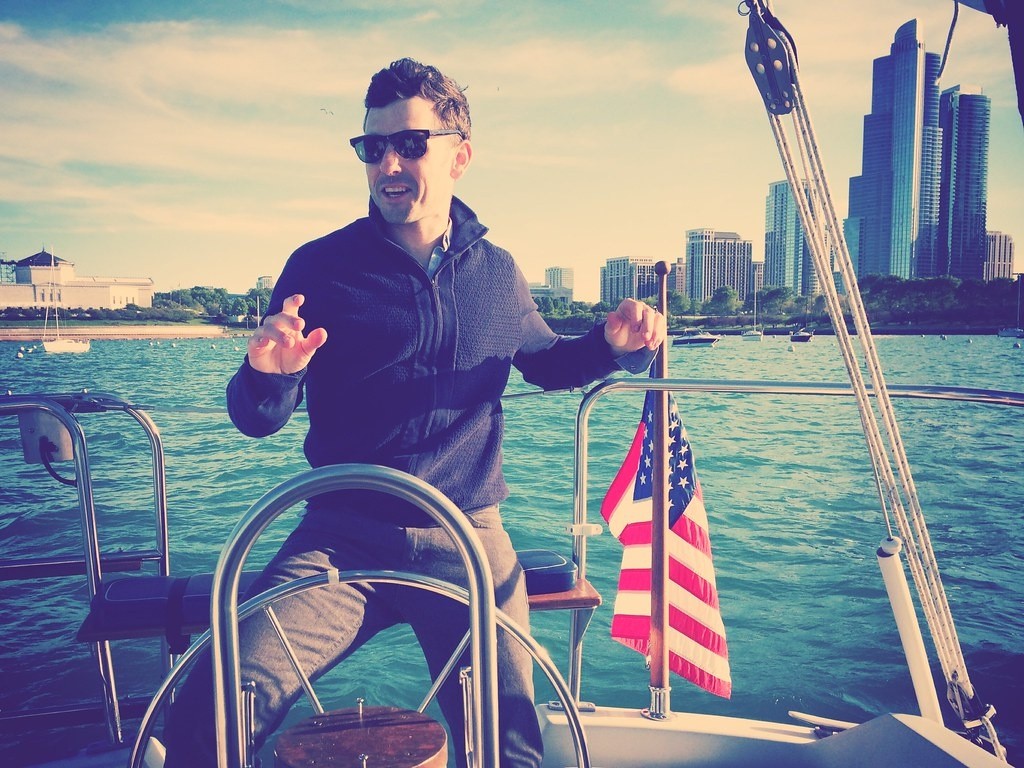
[{"x1": 162, "y1": 58, "x2": 661, "y2": 768}]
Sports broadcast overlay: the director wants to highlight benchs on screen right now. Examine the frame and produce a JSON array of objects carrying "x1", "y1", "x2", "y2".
[{"x1": 74, "y1": 548, "x2": 602, "y2": 685}]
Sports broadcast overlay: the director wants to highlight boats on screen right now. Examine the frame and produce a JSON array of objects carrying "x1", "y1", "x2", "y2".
[{"x1": 671, "y1": 326, "x2": 721, "y2": 348}]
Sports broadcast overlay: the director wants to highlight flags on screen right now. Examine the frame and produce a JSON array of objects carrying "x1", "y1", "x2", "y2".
[{"x1": 600, "y1": 345, "x2": 731, "y2": 698}]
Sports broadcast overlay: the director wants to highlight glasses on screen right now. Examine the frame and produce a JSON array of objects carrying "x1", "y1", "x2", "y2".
[{"x1": 349, "y1": 129, "x2": 464, "y2": 164}]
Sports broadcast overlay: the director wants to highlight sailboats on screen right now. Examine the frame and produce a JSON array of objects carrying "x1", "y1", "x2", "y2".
[
  {"x1": 743, "y1": 270, "x2": 765, "y2": 342},
  {"x1": 790, "y1": 277, "x2": 815, "y2": 342},
  {"x1": 41, "y1": 252, "x2": 90, "y2": 353},
  {"x1": 997, "y1": 275, "x2": 1024, "y2": 338}
]
